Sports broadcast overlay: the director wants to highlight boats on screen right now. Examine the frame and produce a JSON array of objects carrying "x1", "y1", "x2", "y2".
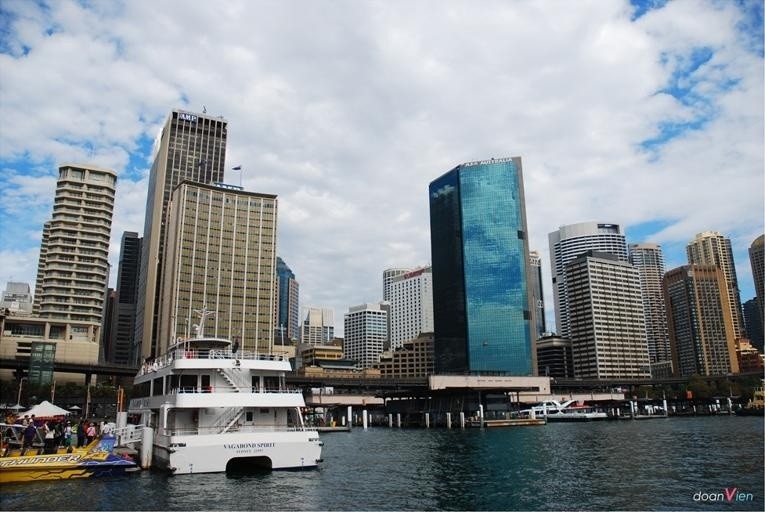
[
  {"x1": 127, "y1": 303, "x2": 326, "y2": 478},
  {"x1": 507, "y1": 397, "x2": 609, "y2": 421},
  {"x1": 0, "y1": 434, "x2": 144, "y2": 483}
]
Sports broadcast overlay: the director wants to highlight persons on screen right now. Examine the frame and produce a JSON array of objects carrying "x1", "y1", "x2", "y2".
[
  {"x1": 304, "y1": 412, "x2": 334, "y2": 426},
  {"x1": 0, "y1": 414, "x2": 138, "y2": 456}
]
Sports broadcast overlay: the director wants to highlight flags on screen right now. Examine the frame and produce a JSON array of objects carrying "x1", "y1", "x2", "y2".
[{"x1": 232, "y1": 166, "x2": 240, "y2": 170}]
